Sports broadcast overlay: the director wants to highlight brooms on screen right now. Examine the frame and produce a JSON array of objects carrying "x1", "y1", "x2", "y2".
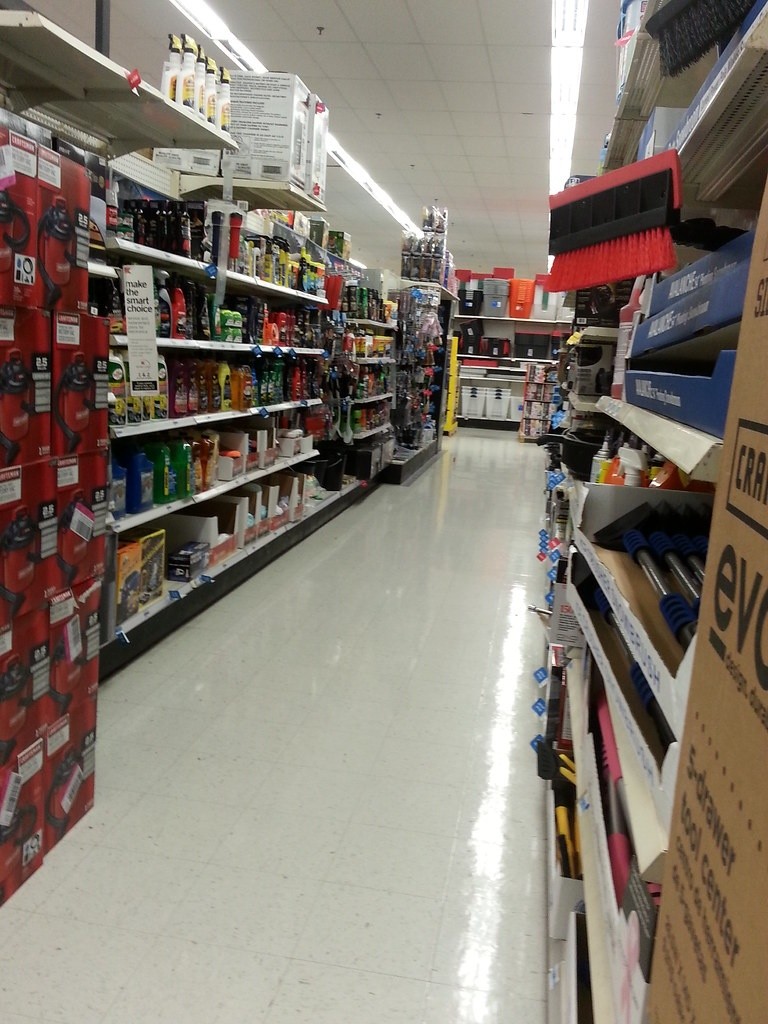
[
  {"x1": 546, "y1": 148, "x2": 751, "y2": 294},
  {"x1": 645, "y1": 0, "x2": 758, "y2": 81}
]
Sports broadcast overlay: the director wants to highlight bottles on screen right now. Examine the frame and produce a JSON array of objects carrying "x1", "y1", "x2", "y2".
[
  {"x1": 610, "y1": 276, "x2": 646, "y2": 397},
  {"x1": 108, "y1": 348, "x2": 259, "y2": 427},
  {"x1": 589, "y1": 442, "x2": 666, "y2": 488},
  {"x1": 127, "y1": 432, "x2": 215, "y2": 513},
  {"x1": 122, "y1": 199, "x2": 191, "y2": 258}
]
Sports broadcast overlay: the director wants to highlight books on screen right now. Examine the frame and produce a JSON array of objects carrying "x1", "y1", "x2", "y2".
[{"x1": 521, "y1": 363, "x2": 557, "y2": 436}]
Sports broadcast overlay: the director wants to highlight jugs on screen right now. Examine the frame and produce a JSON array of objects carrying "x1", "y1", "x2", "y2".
[{"x1": 0, "y1": 187, "x2": 96, "y2": 863}]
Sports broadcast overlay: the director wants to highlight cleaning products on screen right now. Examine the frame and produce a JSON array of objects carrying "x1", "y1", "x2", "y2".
[
  {"x1": 589, "y1": 430, "x2": 666, "y2": 487},
  {"x1": 107, "y1": 348, "x2": 309, "y2": 428},
  {"x1": 112, "y1": 432, "x2": 215, "y2": 521},
  {"x1": 99, "y1": 266, "x2": 324, "y2": 350}
]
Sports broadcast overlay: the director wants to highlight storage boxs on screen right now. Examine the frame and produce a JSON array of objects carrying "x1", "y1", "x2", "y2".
[
  {"x1": 153, "y1": 147, "x2": 221, "y2": 177},
  {"x1": 220, "y1": 71, "x2": 312, "y2": 188},
  {"x1": 97, "y1": 0, "x2": 169, "y2": 90},
  {"x1": 458, "y1": 267, "x2": 574, "y2": 321},
  {"x1": 0, "y1": 127, "x2": 107, "y2": 905},
  {"x1": 461, "y1": 385, "x2": 523, "y2": 419},
  {"x1": 1, "y1": 0, "x2": 96, "y2": 49},
  {"x1": 304, "y1": 93, "x2": 330, "y2": 205}
]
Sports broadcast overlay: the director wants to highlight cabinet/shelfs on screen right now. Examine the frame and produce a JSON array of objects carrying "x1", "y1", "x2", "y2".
[
  {"x1": 453, "y1": 315, "x2": 574, "y2": 423},
  {"x1": 345, "y1": 318, "x2": 396, "y2": 439},
  {"x1": 109, "y1": 236, "x2": 329, "y2": 635}
]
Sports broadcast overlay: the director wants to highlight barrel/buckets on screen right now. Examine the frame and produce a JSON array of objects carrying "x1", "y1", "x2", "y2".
[{"x1": 318, "y1": 453, "x2": 347, "y2": 490}]
